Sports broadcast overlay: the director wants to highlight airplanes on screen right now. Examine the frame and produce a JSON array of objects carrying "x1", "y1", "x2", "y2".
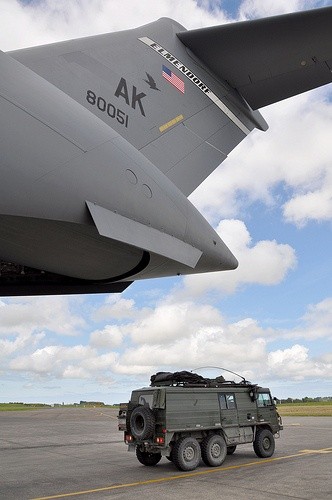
[{"x1": 0, "y1": 0, "x2": 332, "y2": 295}]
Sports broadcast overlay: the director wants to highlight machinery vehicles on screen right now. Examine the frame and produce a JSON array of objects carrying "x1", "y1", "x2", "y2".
[{"x1": 119, "y1": 367, "x2": 285, "y2": 471}]
